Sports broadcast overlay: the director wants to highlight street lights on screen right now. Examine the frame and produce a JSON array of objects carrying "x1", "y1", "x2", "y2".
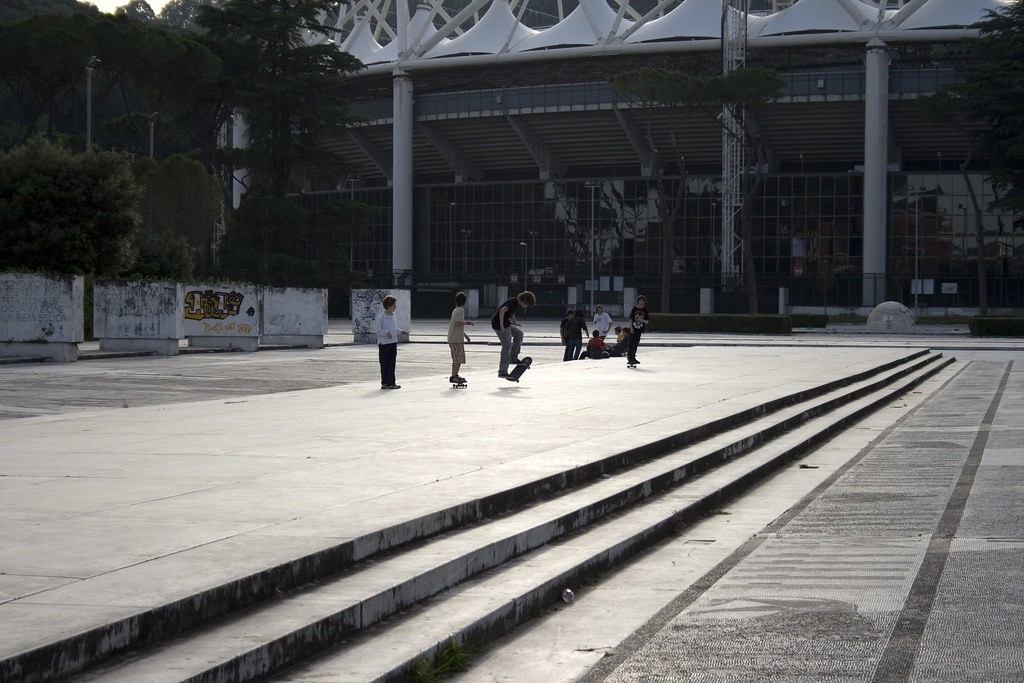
[
  {"x1": 449, "y1": 203, "x2": 456, "y2": 282},
  {"x1": 710, "y1": 203, "x2": 717, "y2": 288},
  {"x1": 520, "y1": 242, "x2": 527, "y2": 290},
  {"x1": 345, "y1": 178, "x2": 361, "y2": 271},
  {"x1": 82, "y1": 55, "x2": 102, "y2": 153},
  {"x1": 461, "y1": 230, "x2": 471, "y2": 288},
  {"x1": 149, "y1": 111, "x2": 160, "y2": 158},
  {"x1": 583, "y1": 179, "x2": 602, "y2": 322}
]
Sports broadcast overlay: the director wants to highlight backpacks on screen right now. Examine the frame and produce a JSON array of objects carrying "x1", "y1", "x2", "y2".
[{"x1": 590, "y1": 343, "x2": 602, "y2": 359}]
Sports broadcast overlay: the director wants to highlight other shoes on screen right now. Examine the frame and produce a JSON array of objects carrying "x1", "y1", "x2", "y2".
[
  {"x1": 627, "y1": 361, "x2": 636, "y2": 364},
  {"x1": 388, "y1": 384, "x2": 399, "y2": 389},
  {"x1": 634, "y1": 358, "x2": 640, "y2": 364},
  {"x1": 381, "y1": 383, "x2": 387, "y2": 389},
  {"x1": 449, "y1": 375, "x2": 467, "y2": 383},
  {"x1": 498, "y1": 373, "x2": 510, "y2": 378},
  {"x1": 509, "y1": 358, "x2": 527, "y2": 365}
]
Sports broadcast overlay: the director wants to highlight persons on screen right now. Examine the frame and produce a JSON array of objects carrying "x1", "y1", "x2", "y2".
[
  {"x1": 376, "y1": 295, "x2": 410, "y2": 390},
  {"x1": 490, "y1": 291, "x2": 536, "y2": 379},
  {"x1": 561, "y1": 294, "x2": 649, "y2": 364},
  {"x1": 447, "y1": 291, "x2": 474, "y2": 384}
]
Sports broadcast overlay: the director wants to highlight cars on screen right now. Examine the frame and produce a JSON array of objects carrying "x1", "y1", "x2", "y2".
[
  {"x1": 931, "y1": 254, "x2": 1024, "y2": 308},
  {"x1": 804, "y1": 250, "x2": 899, "y2": 309}
]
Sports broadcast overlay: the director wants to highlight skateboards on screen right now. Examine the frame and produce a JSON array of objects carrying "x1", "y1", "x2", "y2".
[
  {"x1": 506, "y1": 357, "x2": 532, "y2": 383},
  {"x1": 627, "y1": 362, "x2": 640, "y2": 368},
  {"x1": 450, "y1": 381, "x2": 467, "y2": 388}
]
